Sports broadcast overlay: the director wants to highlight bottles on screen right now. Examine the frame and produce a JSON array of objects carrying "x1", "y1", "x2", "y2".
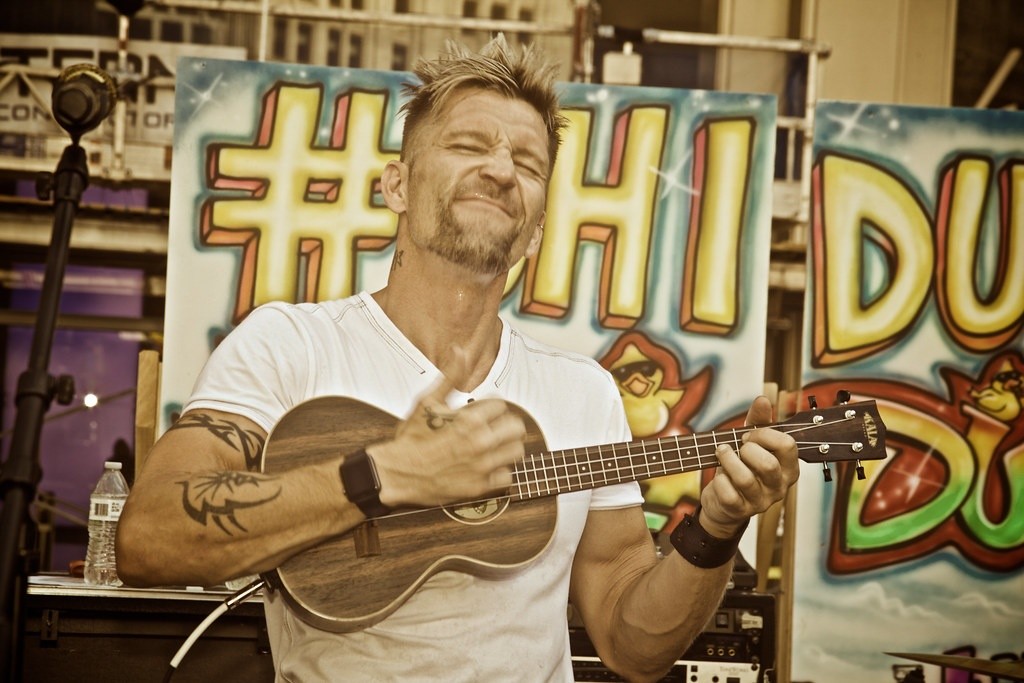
[{"x1": 84, "y1": 462, "x2": 130, "y2": 587}]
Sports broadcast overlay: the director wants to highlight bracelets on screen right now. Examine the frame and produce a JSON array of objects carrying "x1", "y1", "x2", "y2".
[{"x1": 670, "y1": 505, "x2": 750, "y2": 568}]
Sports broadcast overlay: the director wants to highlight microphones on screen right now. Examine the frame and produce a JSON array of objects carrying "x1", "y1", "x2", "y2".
[{"x1": 53, "y1": 65, "x2": 117, "y2": 124}]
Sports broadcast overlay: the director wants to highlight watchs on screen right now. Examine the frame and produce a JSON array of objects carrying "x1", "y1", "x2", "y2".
[{"x1": 338, "y1": 450, "x2": 388, "y2": 519}]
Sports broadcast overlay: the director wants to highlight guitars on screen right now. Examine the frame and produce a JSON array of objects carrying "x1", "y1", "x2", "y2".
[{"x1": 261, "y1": 390, "x2": 888, "y2": 636}]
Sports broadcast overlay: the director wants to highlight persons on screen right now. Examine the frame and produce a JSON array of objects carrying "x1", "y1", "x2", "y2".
[{"x1": 115, "y1": 31, "x2": 799, "y2": 683}]
